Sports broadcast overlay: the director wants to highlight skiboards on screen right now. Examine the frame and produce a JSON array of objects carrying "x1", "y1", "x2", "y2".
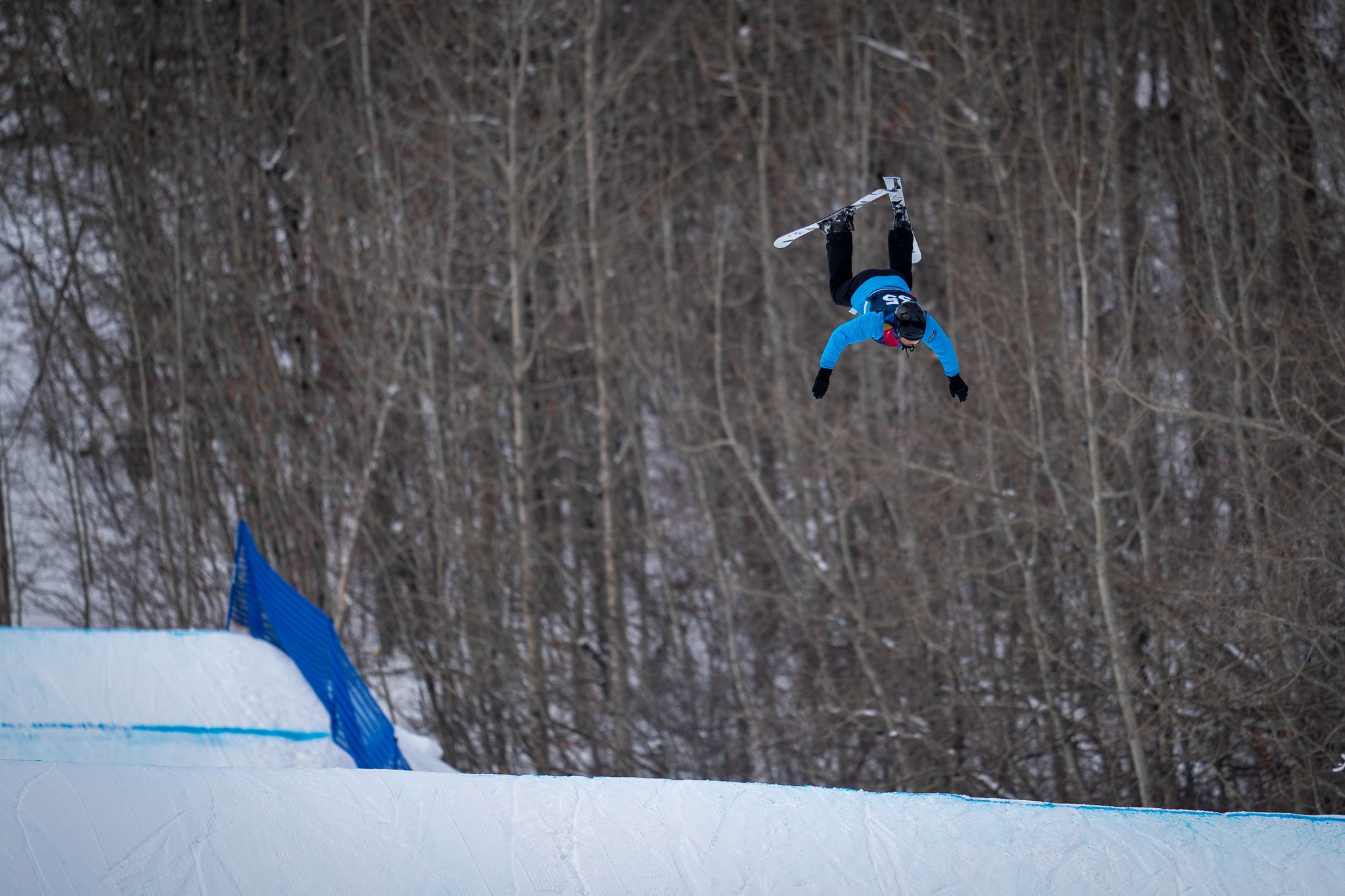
[{"x1": 773, "y1": 176, "x2": 922, "y2": 264}]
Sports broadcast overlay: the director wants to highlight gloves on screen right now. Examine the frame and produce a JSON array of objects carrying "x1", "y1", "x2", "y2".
[
  {"x1": 812, "y1": 368, "x2": 833, "y2": 399},
  {"x1": 946, "y1": 373, "x2": 968, "y2": 403}
]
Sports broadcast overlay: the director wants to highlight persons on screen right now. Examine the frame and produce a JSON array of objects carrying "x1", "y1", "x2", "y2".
[{"x1": 811, "y1": 203, "x2": 969, "y2": 403}]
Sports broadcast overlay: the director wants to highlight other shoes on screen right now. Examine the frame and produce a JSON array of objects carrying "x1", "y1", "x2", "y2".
[
  {"x1": 892, "y1": 212, "x2": 907, "y2": 230},
  {"x1": 824, "y1": 216, "x2": 849, "y2": 235}
]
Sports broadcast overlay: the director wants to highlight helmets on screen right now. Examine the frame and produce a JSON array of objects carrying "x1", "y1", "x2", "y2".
[{"x1": 895, "y1": 301, "x2": 926, "y2": 341}]
